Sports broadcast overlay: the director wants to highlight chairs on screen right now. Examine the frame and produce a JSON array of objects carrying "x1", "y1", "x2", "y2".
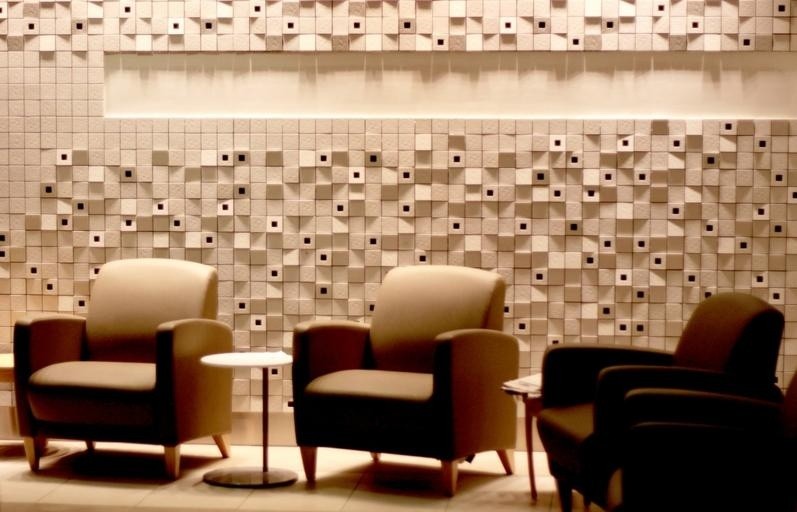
[
  {"x1": 291, "y1": 262, "x2": 523, "y2": 494},
  {"x1": 13, "y1": 255, "x2": 232, "y2": 482}
]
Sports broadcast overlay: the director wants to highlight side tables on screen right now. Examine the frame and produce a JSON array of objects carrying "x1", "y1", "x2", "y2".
[
  {"x1": 507, "y1": 388, "x2": 547, "y2": 502},
  {"x1": 199, "y1": 352, "x2": 297, "y2": 490}
]
